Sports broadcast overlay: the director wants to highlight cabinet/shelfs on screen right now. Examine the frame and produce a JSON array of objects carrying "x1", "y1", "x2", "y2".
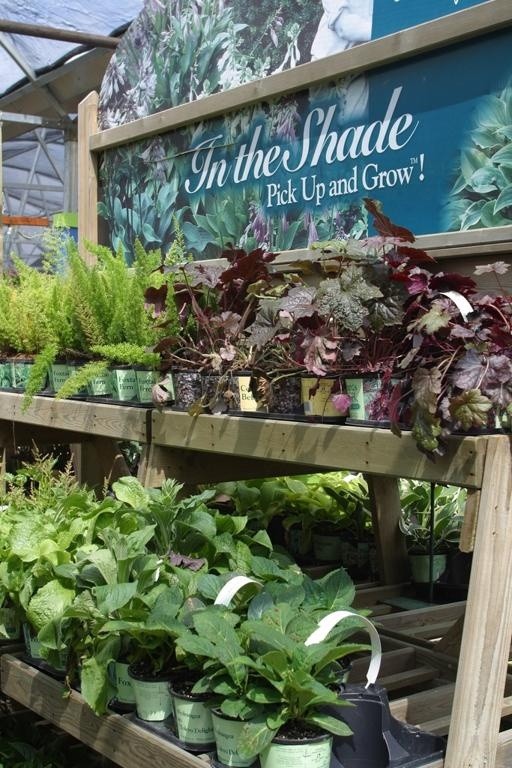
[
  {"x1": 2, "y1": 438, "x2": 470, "y2": 768},
  {"x1": 0, "y1": 384, "x2": 512, "y2": 768},
  {"x1": 0, "y1": 198, "x2": 512, "y2": 461}
]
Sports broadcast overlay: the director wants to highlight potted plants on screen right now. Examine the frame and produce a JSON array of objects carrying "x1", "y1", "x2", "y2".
[
  {"x1": 42, "y1": 477, "x2": 111, "y2": 675},
  {"x1": 121, "y1": 488, "x2": 178, "y2": 722},
  {"x1": 265, "y1": 260, "x2": 302, "y2": 409},
  {"x1": 107, "y1": 238, "x2": 137, "y2": 402},
  {"x1": 0, "y1": 244, "x2": 15, "y2": 391},
  {"x1": 168, "y1": 514, "x2": 261, "y2": 768},
  {"x1": 71, "y1": 232, "x2": 112, "y2": 398},
  {"x1": 248, "y1": 471, "x2": 457, "y2": 587},
  {"x1": 162, "y1": 249, "x2": 203, "y2": 411},
  {"x1": 201, "y1": 239, "x2": 233, "y2": 411},
  {"x1": 99, "y1": 479, "x2": 137, "y2": 707},
  {"x1": 337, "y1": 241, "x2": 409, "y2": 424},
  {"x1": 133, "y1": 247, "x2": 166, "y2": 405},
  {"x1": 203, "y1": 568, "x2": 383, "y2": 768},
  {"x1": 294, "y1": 239, "x2": 350, "y2": 424},
  {"x1": 230, "y1": 246, "x2": 269, "y2": 414},
  {"x1": 12, "y1": 250, "x2": 48, "y2": 394},
  {"x1": 37, "y1": 214, "x2": 79, "y2": 400}
]
